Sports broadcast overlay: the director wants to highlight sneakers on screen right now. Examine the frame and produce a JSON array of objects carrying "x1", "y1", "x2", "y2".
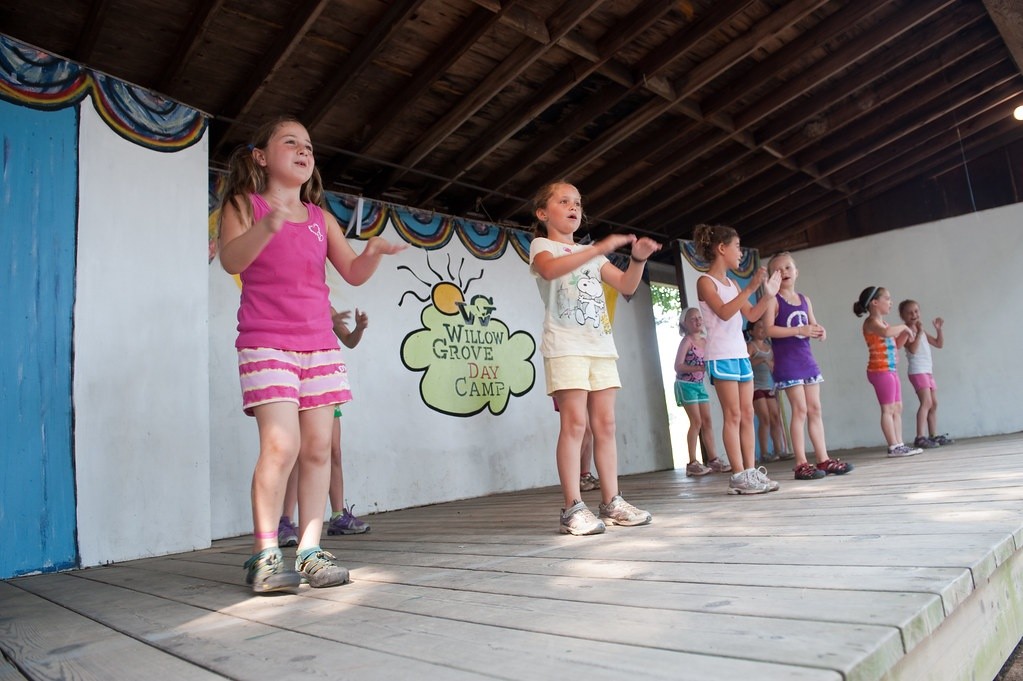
[
  {"x1": 686, "y1": 462, "x2": 711, "y2": 477},
  {"x1": 277, "y1": 516, "x2": 298, "y2": 546},
  {"x1": 559, "y1": 500, "x2": 604, "y2": 535},
  {"x1": 707, "y1": 459, "x2": 732, "y2": 471},
  {"x1": 794, "y1": 463, "x2": 825, "y2": 478},
  {"x1": 749, "y1": 466, "x2": 779, "y2": 491},
  {"x1": 294, "y1": 551, "x2": 349, "y2": 587},
  {"x1": 901, "y1": 445, "x2": 923, "y2": 454},
  {"x1": 914, "y1": 437, "x2": 939, "y2": 448},
  {"x1": 580, "y1": 476, "x2": 600, "y2": 490},
  {"x1": 243, "y1": 550, "x2": 300, "y2": 592},
  {"x1": 598, "y1": 491, "x2": 651, "y2": 526},
  {"x1": 887, "y1": 447, "x2": 916, "y2": 457},
  {"x1": 930, "y1": 433, "x2": 954, "y2": 444},
  {"x1": 326, "y1": 503, "x2": 370, "y2": 535},
  {"x1": 817, "y1": 458, "x2": 852, "y2": 474},
  {"x1": 728, "y1": 470, "x2": 768, "y2": 495}
]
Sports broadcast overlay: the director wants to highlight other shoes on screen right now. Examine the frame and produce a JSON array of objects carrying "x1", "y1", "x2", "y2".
[
  {"x1": 759, "y1": 452, "x2": 778, "y2": 463},
  {"x1": 778, "y1": 452, "x2": 797, "y2": 459}
]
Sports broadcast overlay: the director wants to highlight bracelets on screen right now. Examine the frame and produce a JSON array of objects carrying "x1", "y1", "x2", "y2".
[{"x1": 631, "y1": 255, "x2": 647, "y2": 264}]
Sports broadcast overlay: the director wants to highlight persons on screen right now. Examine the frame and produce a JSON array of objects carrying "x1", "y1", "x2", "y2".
[
  {"x1": 693, "y1": 221, "x2": 783, "y2": 495},
  {"x1": 278, "y1": 307, "x2": 371, "y2": 549},
  {"x1": 743, "y1": 317, "x2": 795, "y2": 461},
  {"x1": 897, "y1": 299, "x2": 955, "y2": 448},
  {"x1": 673, "y1": 307, "x2": 732, "y2": 476},
  {"x1": 852, "y1": 285, "x2": 923, "y2": 457},
  {"x1": 763, "y1": 249, "x2": 854, "y2": 479},
  {"x1": 217, "y1": 113, "x2": 411, "y2": 592},
  {"x1": 552, "y1": 395, "x2": 601, "y2": 491},
  {"x1": 527, "y1": 180, "x2": 664, "y2": 537}
]
